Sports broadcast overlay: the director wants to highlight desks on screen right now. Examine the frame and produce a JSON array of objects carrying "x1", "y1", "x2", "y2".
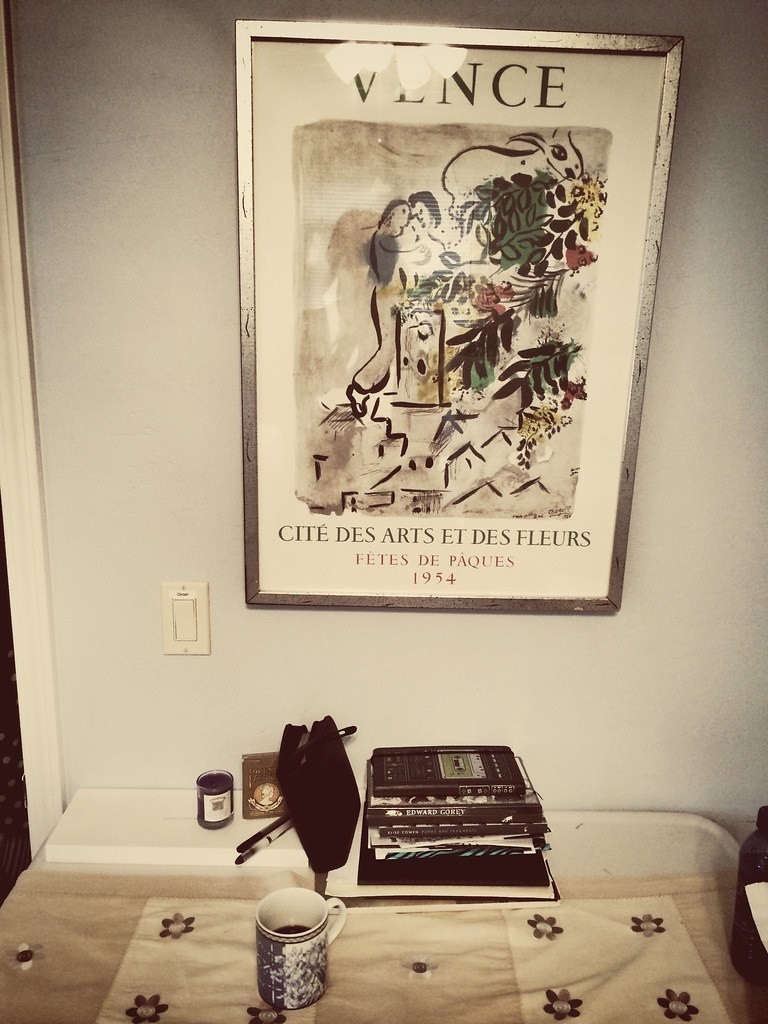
[{"x1": 0, "y1": 788, "x2": 768, "y2": 1024}]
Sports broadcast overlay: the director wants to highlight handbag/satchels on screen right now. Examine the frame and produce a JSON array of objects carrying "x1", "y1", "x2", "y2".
[{"x1": 276, "y1": 719, "x2": 361, "y2": 872}]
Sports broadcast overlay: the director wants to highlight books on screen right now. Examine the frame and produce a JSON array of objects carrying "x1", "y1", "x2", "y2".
[
  {"x1": 356, "y1": 746, "x2": 563, "y2": 901},
  {"x1": 241, "y1": 751, "x2": 288, "y2": 819}
]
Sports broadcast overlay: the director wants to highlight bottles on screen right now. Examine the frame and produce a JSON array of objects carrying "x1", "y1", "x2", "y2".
[{"x1": 730, "y1": 805, "x2": 768, "y2": 986}]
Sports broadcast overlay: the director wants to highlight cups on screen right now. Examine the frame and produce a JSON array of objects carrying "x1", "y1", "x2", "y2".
[
  {"x1": 196, "y1": 770, "x2": 235, "y2": 829},
  {"x1": 256, "y1": 887, "x2": 347, "y2": 1011}
]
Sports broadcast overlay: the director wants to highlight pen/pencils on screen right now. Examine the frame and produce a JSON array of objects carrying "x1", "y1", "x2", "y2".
[
  {"x1": 235, "y1": 815, "x2": 293, "y2": 865},
  {"x1": 339, "y1": 725, "x2": 357, "y2": 737}
]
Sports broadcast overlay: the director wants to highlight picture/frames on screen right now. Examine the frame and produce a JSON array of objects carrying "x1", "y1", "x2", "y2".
[{"x1": 233, "y1": 17, "x2": 684, "y2": 612}]
